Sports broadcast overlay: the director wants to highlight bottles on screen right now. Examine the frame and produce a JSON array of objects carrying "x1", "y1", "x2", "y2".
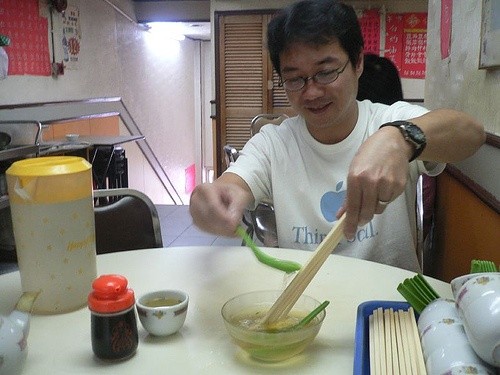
[{"x1": 87, "y1": 274, "x2": 139, "y2": 364}]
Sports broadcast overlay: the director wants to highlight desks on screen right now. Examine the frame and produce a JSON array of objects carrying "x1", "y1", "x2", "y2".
[{"x1": 0, "y1": 245, "x2": 458, "y2": 375}]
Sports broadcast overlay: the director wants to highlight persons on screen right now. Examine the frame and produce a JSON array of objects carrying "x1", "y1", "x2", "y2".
[
  {"x1": 189, "y1": 0, "x2": 486, "y2": 276},
  {"x1": 357, "y1": 53, "x2": 436, "y2": 255}
]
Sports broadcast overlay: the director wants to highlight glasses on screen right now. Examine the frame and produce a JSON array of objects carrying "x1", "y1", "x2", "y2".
[{"x1": 278, "y1": 56, "x2": 350, "y2": 91}]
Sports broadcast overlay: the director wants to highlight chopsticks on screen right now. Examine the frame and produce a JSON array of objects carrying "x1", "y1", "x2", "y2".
[
  {"x1": 368, "y1": 306, "x2": 428, "y2": 375},
  {"x1": 260, "y1": 212, "x2": 348, "y2": 326}
]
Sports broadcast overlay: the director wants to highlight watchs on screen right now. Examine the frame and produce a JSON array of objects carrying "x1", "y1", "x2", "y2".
[{"x1": 379, "y1": 120, "x2": 427, "y2": 163}]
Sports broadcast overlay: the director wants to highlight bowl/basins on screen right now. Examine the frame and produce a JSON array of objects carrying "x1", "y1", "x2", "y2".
[
  {"x1": 65, "y1": 134, "x2": 79, "y2": 141},
  {"x1": 417, "y1": 271, "x2": 500, "y2": 375},
  {"x1": 0, "y1": 316, "x2": 29, "y2": 375},
  {"x1": 136, "y1": 289, "x2": 190, "y2": 337},
  {"x1": 221, "y1": 289, "x2": 327, "y2": 362}
]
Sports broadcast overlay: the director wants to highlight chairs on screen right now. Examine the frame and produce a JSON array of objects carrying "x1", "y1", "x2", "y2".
[{"x1": 91, "y1": 188, "x2": 163, "y2": 254}]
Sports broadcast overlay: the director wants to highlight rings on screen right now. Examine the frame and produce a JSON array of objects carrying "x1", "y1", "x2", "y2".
[{"x1": 379, "y1": 200, "x2": 390, "y2": 206}]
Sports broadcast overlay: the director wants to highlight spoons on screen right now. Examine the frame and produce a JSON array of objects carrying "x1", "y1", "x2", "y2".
[
  {"x1": 235, "y1": 225, "x2": 301, "y2": 274},
  {"x1": 263, "y1": 300, "x2": 330, "y2": 334}
]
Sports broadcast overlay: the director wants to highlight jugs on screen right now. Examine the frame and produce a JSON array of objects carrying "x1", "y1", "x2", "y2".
[{"x1": 4, "y1": 155, "x2": 98, "y2": 314}]
